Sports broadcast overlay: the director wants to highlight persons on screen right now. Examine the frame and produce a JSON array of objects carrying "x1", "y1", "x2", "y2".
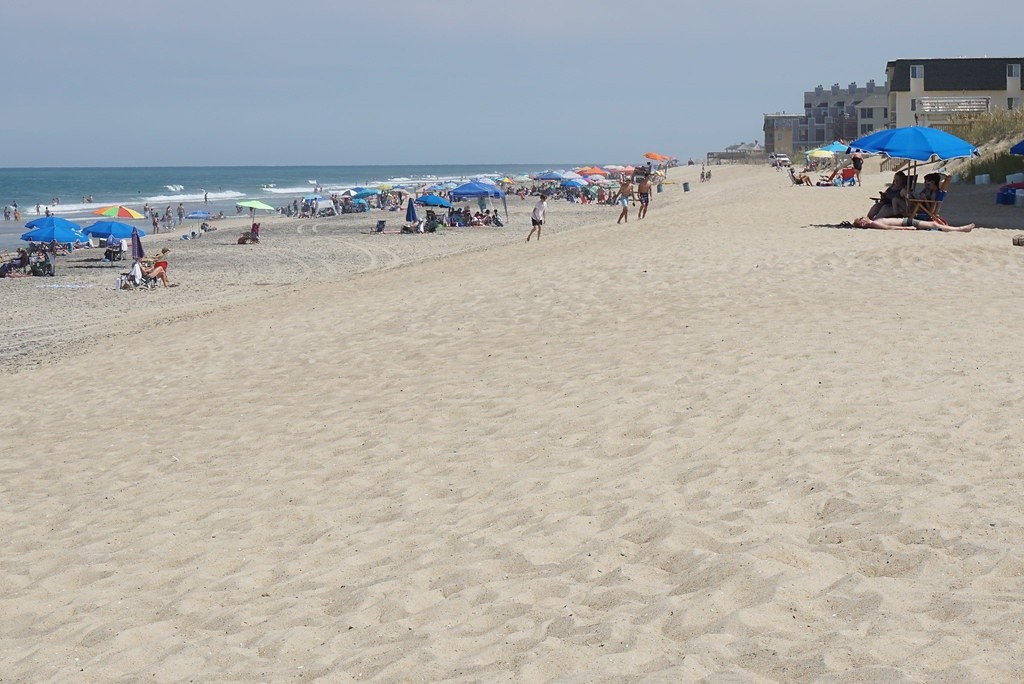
[
  {"x1": 509, "y1": 172, "x2": 654, "y2": 241},
  {"x1": 0, "y1": 239, "x2": 176, "y2": 289},
  {"x1": 143, "y1": 192, "x2": 259, "y2": 245},
  {"x1": 3, "y1": 195, "x2": 95, "y2": 221},
  {"x1": 400, "y1": 207, "x2": 504, "y2": 235},
  {"x1": 276, "y1": 191, "x2": 402, "y2": 218},
  {"x1": 774, "y1": 150, "x2": 864, "y2": 188},
  {"x1": 642, "y1": 158, "x2": 713, "y2": 183},
  {"x1": 854, "y1": 172, "x2": 976, "y2": 232}
]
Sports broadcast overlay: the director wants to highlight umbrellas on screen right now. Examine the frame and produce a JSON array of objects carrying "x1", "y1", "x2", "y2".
[
  {"x1": 306, "y1": 183, "x2": 405, "y2": 212},
  {"x1": 850, "y1": 126, "x2": 981, "y2": 216},
  {"x1": 21, "y1": 205, "x2": 145, "y2": 267},
  {"x1": 406, "y1": 198, "x2": 417, "y2": 225},
  {"x1": 494, "y1": 165, "x2": 637, "y2": 187},
  {"x1": 237, "y1": 201, "x2": 274, "y2": 224},
  {"x1": 416, "y1": 194, "x2": 451, "y2": 208},
  {"x1": 643, "y1": 152, "x2": 676, "y2": 164},
  {"x1": 804, "y1": 141, "x2": 849, "y2": 170}
]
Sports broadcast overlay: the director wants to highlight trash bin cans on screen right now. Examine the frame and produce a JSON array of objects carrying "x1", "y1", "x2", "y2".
[{"x1": 683, "y1": 182, "x2": 690, "y2": 192}]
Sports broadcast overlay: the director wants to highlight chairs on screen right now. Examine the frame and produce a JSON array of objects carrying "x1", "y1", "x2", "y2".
[
  {"x1": 120, "y1": 273, "x2": 157, "y2": 288},
  {"x1": 820, "y1": 168, "x2": 839, "y2": 182},
  {"x1": 106, "y1": 239, "x2": 126, "y2": 260},
  {"x1": 241, "y1": 223, "x2": 260, "y2": 244},
  {"x1": 870, "y1": 172, "x2": 952, "y2": 226},
  {"x1": 426, "y1": 210, "x2": 435, "y2": 220},
  {"x1": 404, "y1": 221, "x2": 423, "y2": 234},
  {"x1": 840, "y1": 167, "x2": 855, "y2": 187},
  {"x1": 0, "y1": 248, "x2": 23, "y2": 275},
  {"x1": 370, "y1": 220, "x2": 386, "y2": 234},
  {"x1": 787, "y1": 169, "x2": 804, "y2": 186}
]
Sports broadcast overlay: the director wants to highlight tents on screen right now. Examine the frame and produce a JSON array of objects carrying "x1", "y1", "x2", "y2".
[{"x1": 446, "y1": 181, "x2": 507, "y2": 222}]
